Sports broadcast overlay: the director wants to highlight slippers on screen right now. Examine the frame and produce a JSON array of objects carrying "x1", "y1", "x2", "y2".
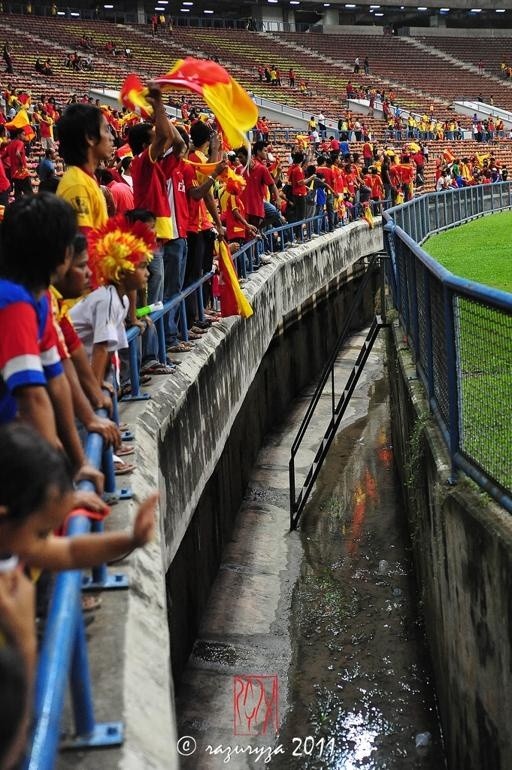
[
  {"x1": 117, "y1": 445, "x2": 134, "y2": 456},
  {"x1": 111, "y1": 453, "x2": 136, "y2": 473}
]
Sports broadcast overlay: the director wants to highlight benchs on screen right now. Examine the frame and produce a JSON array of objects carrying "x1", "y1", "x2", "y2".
[{"x1": 0, "y1": 12, "x2": 512, "y2": 213}]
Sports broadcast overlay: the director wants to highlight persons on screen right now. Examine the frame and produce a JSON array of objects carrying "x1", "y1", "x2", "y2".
[
  {"x1": 252, "y1": 52, "x2": 511, "y2": 269},
  {"x1": 1, "y1": 1, "x2": 253, "y2": 767}
]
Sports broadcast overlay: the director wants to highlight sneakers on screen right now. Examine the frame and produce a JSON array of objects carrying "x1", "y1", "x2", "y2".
[
  {"x1": 253, "y1": 253, "x2": 273, "y2": 269},
  {"x1": 169, "y1": 310, "x2": 220, "y2": 352},
  {"x1": 287, "y1": 231, "x2": 327, "y2": 248},
  {"x1": 141, "y1": 357, "x2": 182, "y2": 384}
]
